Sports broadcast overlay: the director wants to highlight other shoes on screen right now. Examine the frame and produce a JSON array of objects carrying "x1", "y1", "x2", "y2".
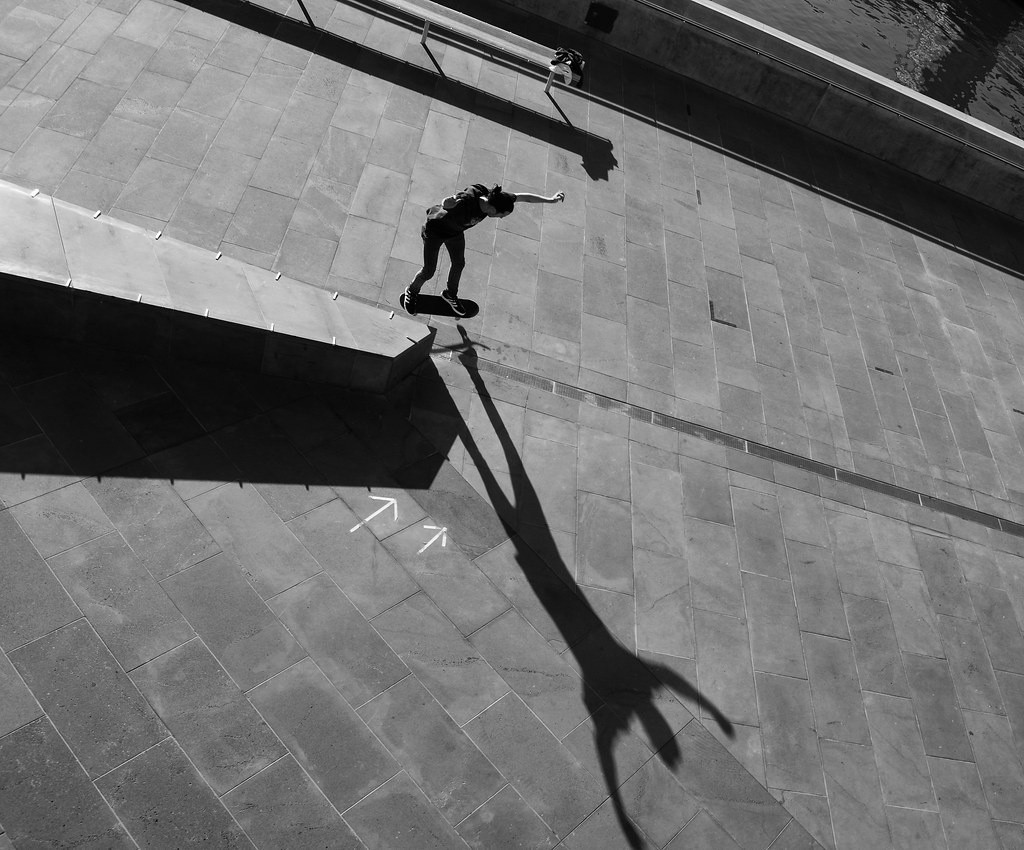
[
  {"x1": 404, "y1": 286, "x2": 417, "y2": 317},
  {"x1": 440, "y1": 288, "x2": 466, "y2": 316}
]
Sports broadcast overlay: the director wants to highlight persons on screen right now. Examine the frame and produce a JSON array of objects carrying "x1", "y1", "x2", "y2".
[{"x1": 404, "y1": 184, "x2": 565, "y2": 316}]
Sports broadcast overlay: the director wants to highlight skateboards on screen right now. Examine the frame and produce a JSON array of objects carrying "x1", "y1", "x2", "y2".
[{"x1": 399, "y1": 292, "x2": 479, "y2": 320}]
